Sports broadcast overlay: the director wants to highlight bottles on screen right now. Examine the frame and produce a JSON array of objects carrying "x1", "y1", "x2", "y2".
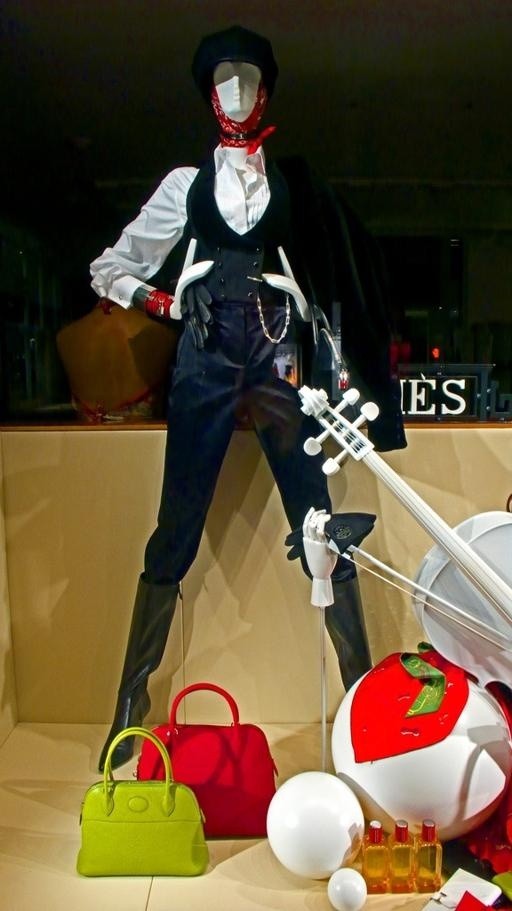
[
  {"x1": 387, "y1": 820, "x2": 414, "y2": 895},
  {"x1": 414, "y1": 819, "x2": 444, "y2": 895},
  {"x1": 360, "y1": 820, "x2": 387, "y2": 895}
]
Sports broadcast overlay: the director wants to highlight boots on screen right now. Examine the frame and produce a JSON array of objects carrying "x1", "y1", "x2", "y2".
[
  {"x1": 313, "y1": 577, "x2": 373, "y2": 696},
  {"x1": 97, "y1": 572, "x2": 182, "y2": 772}
]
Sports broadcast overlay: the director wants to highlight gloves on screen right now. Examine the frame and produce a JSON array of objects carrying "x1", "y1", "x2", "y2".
[
  {"x1": 181, "y1": 283, "x2": 215, "y2": 353},
  {"x1": 285, "y1": 511, "x2": 377, "y2": 562}
]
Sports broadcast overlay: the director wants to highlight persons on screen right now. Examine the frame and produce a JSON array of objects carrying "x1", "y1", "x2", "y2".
[{"x1": 88, "y1": 27, "x2": 407, "y2": 775}]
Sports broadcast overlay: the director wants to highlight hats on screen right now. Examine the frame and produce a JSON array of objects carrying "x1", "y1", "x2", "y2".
[{"x1": 190, "y1": 26, "x2": 280, "y2": 100}]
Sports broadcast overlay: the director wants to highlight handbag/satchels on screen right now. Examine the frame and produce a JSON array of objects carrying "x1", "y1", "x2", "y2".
[
  {"x1": 74, "y1": 727, "x2": 209, "y2": 878},
  {"x1": 138, "y1": 680, "x2": 277, "y2": 841}
]
